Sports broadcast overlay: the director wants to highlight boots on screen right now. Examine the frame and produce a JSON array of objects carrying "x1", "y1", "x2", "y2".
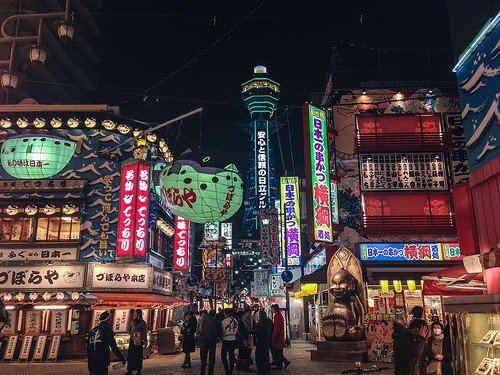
[
  {"x1": 237, "y1": 359, "x2": 253, "y2": 372},
  {"x1": 181, "y1": 361, "x2": 191, "y2": 368}
]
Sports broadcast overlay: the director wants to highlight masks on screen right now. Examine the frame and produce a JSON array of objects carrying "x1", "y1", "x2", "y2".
[{"x1": 433, "y1": 329, "x2": 441, "y2": 335}]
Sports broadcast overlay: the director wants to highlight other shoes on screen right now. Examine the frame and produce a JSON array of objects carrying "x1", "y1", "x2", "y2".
[
  {"x1": 124, "y1": 372, "x2": 132, "y2": 375},
  {"x1": 136, "y1": 373, "x2": 141, "y2": 375},
  {"x1": 272, "y1": 367, "x2": 283, "y2": 370},
  {"x1": 284, "y1": 361, "x2": 291, "y2": 369}
]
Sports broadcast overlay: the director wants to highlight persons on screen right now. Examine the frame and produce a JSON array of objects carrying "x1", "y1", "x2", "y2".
[
  {"x1": 321, "y1": 268, "x2": 365, "y2": 342},
  {"x1": 393, "y1": 306, "x2": 458, "y2": 375},
  {"x1": 124, "y1": 309, "x2": 148, "y2": 375},
  {"x1": 181, "y1": 304, "x2": 291, "y2": 375},
  {"x1": 86, "y1": 311, "x2": 126, "y2": 375}
]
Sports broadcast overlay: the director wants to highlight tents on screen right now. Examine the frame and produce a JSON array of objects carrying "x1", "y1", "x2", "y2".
[{"x1": 422, "y1": 248, "x2": 500, "y2": 340}]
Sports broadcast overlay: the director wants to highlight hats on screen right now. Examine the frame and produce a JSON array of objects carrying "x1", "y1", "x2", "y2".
[
  {"x1": 272, "y1": 304, "x2": 279, "y2": 312},
  {"x1": 99, "y1": 312, "x2": 110, "y2": 322}
]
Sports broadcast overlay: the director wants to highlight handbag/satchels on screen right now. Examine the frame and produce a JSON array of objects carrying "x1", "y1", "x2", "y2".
[{"x1": 243, "y1": 336, "x2": 253, "y2": 349}]
